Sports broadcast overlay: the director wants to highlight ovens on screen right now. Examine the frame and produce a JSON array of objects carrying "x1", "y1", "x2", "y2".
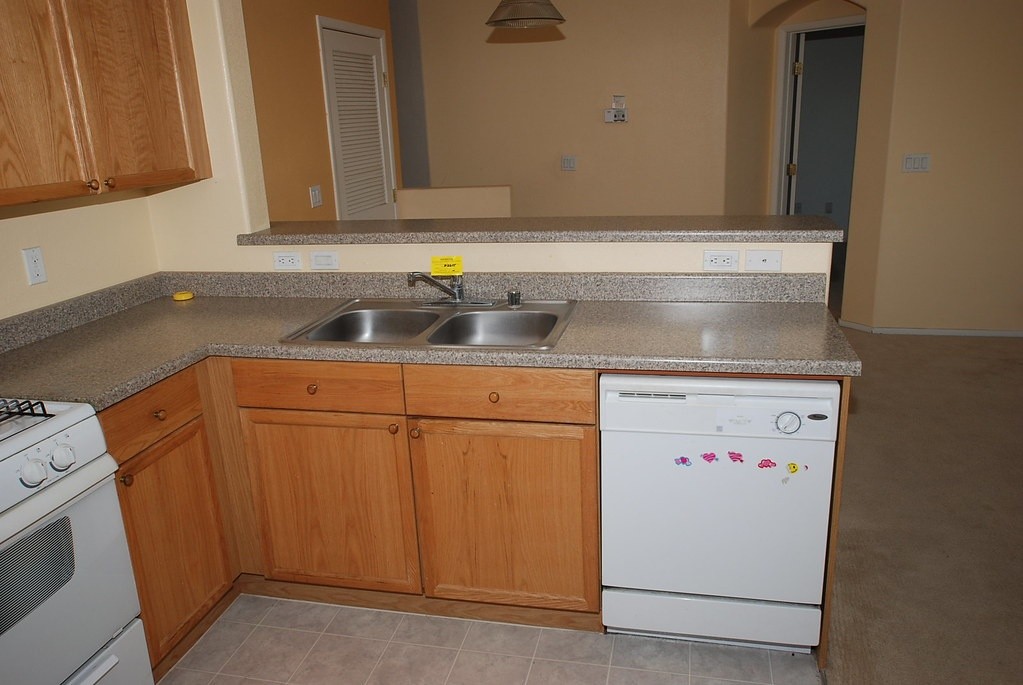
[{"x1": 0, "y1": 449, "x2": 162, "y2": 684}]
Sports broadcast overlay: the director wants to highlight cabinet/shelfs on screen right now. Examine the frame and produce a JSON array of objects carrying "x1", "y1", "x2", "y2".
[
  {"x1": 0, "y1": 0, "x2": 213, "y2": 221},
  {"x1": 95, "y1": 357, "x2": 601, "y2": 685}
]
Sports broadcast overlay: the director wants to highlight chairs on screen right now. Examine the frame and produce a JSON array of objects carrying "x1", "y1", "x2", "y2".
[{"x1": 393, "y1": 184, "x2": 511, "y2": 218}]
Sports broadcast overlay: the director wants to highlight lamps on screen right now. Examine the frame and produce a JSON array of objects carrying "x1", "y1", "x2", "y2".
[{"x1": 485, "y1": 0, "x2": 566, "y2": 29}]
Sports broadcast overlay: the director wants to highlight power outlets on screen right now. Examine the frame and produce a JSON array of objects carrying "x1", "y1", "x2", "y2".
[
  {"x1": 703, "y1": 250, "x2": 740, "y2": 273},
  {"x1": 22, "y1": 246, "x2": 47, "y2": 285},
  {"x1": 272, "y1": 251, "x2": 301, "y2": 270}
]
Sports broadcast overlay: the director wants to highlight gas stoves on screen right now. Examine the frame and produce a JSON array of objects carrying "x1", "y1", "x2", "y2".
[{"x1": 1, "y1": 391, "x2": 111, "y2": 517}]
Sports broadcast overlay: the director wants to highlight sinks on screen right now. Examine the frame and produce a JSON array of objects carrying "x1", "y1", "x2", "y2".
[
  {"x1": 277, "y1": 310, "x2": 439, "y2": 344},
  {"x1": 429, "y1": 311, "x2": 557, "y2": 350}
]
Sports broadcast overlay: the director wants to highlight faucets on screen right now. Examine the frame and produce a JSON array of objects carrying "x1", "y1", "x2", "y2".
[{"x1": 407, "y1": 269, "x2": 464, "y2": 302}]
